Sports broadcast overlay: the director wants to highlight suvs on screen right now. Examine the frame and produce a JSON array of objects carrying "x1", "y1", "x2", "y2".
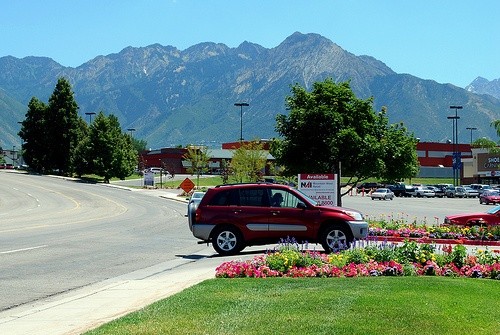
[{"x1": 188, "y1": 182, "x2": 370, "y2": 255}]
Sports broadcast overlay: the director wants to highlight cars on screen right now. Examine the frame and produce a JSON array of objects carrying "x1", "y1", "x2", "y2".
[
  {"x1": 371, "y1": 188, "x2": 395, "y2": 200},
  {"x1": 356, "y1": 183, "x2": 500, "y2": 198},
  {"x1": 479, "y1": 191, "x2": 500, "y2": 205},
  {"x1": 186, "y1": 190, "x2": 206, "y2": 208},
  {"x1": 148, "y1": 167, "x2": 168, "y2": 174}
]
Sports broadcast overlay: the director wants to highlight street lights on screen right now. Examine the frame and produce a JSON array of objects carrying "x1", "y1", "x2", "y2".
[
  {"x1": 12, "y1": 146, "x2": 16, "y2": 166},
  {"x1": 466, "y1": 127, "x2": 477, "y2": 145},
  {"x1": 450, "y1": 105, "x2": 463, "y2": 186},
  {"x1": 127, "y1": 129, "x2": 136, "y2": 137},
  {"x1": 447, "y1": 116, "x2": 460, "y2": 186},
  {"x1": 233, "y1": 103, "x2": 249, "y2": 143},
  {"x1": 84, "y1": 112, "x2": 96, "y2": 124}
]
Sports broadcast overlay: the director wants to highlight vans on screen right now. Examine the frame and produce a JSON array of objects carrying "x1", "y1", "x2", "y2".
[{"x1": 0, "y1": 163, "x2": 15, "y2": 169}]
